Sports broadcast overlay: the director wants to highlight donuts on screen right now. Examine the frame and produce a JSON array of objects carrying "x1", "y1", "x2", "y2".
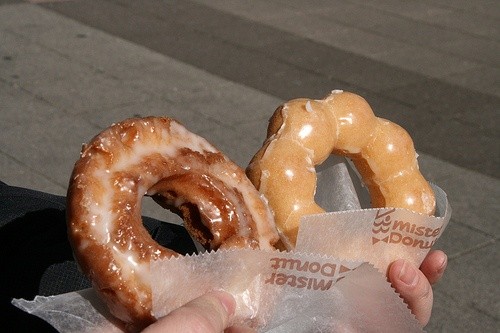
[
  {"x1": 66, "y1": 114, "x2": 279, "y2": 326},
  {"x1": 247, "y1": 87, "x2": 436, "y2": 252}
]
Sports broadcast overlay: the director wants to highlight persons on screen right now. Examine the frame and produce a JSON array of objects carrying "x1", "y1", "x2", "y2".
[{"x1": 84, "y1": 236, "x2": 448, "y2": 332}]
